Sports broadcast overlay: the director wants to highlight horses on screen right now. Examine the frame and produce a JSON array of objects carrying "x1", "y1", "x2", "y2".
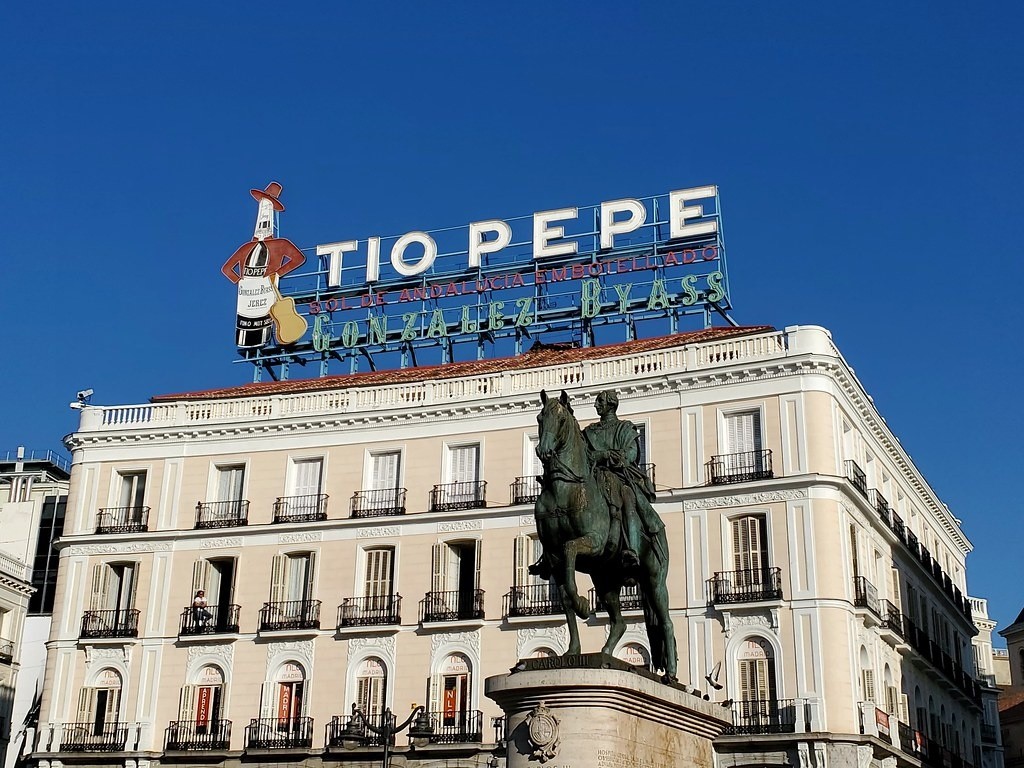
[{"x1": 535, "y1": 390, "x2": 677, "y2": 679}]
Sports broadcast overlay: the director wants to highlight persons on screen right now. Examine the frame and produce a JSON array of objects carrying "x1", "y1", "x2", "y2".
[
  {"x1": 194, "y1": 590, "x2": 213, "y2": 630},
  {"x1": 525, "y1": 389, "x2": 646, "y2": 579}
]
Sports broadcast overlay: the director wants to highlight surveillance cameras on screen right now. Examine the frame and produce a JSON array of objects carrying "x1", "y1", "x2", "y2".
[{"x1": 76, "y1": 389, "x2": 95, "y2": 399}]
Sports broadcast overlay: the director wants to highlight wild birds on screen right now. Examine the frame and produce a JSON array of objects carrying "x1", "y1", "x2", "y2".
[{"x1": 705, "y1": 661, "x2": 723, "y2": 690}]
